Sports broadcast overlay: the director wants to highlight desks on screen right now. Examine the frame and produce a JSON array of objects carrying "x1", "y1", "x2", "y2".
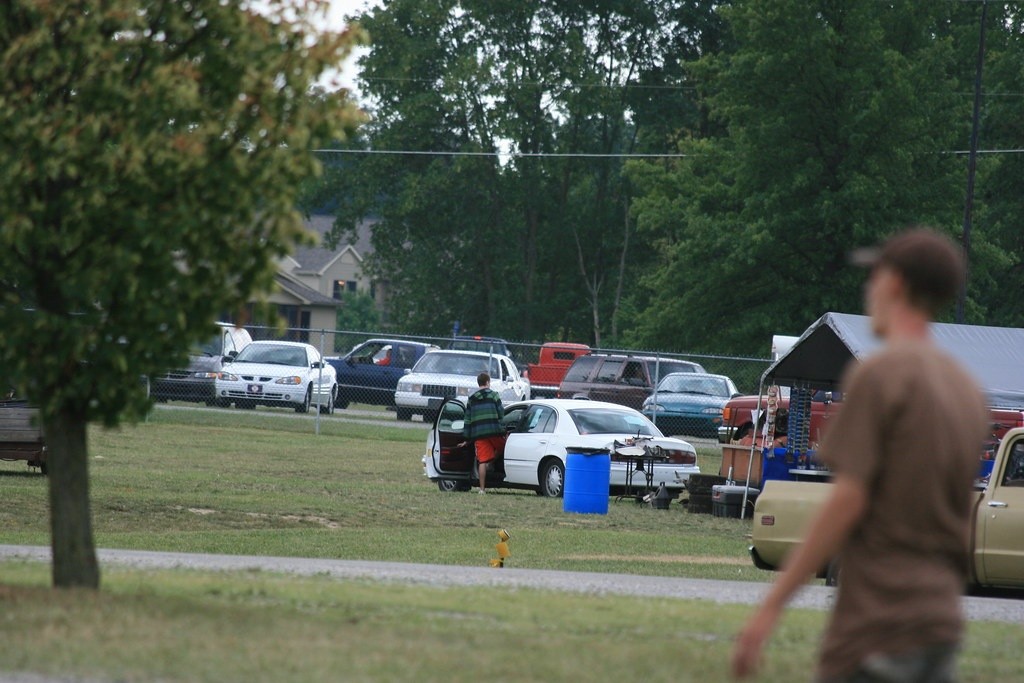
[
  {"x1": 787, "y1": 468, "x2": 833, "y2": 481},
  {"x1": 615, "y1": 455, "x2": 670, "y2": 508}
]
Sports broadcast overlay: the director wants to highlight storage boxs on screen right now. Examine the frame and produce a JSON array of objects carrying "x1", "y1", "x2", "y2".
[
  {"x1": 688, "y1": 494, "x2": 712, "y2": 513},
  {"x1": 688, "y1": 473, "x2": 734, "y2": 495},
  {"x1": 712, "y1": 485, "x2": 760, "y2": 519}
]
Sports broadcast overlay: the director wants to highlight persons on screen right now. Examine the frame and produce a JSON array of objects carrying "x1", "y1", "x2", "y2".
[
  {"x1": 377, "y1": 349, "x2": 391, "y2": 366},
  {"x1": 726, "y1": 231, "x2": 988, "y2": 683},
  {"x1": 456, "y1": 374, "x2": 509, "y2": 494}
]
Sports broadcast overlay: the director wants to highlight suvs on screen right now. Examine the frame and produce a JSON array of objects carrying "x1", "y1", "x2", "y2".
[
  {"x1": 558, "y1": 351, "x2": 709, "y2": 412},
  {"x1": 324, "y1": 339, "x2": 441, "y2": 409}
]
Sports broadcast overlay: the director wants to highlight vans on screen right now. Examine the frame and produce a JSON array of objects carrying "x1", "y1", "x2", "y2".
[{"x1": 139, "y1": 322, "x2": 253, "y2": 406}]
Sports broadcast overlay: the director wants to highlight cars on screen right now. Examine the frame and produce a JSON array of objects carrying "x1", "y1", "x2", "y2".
[
  {"x1": 642, "y1": 372, "x2": 740, "y2": 437},
  {"x1": 423, "y1": 399, "x2": 700, "y2": 497},
  {"x1": 215, "y1": 341, "x2": 338, "y2": 413},
  {"x1": 394, "y1": 336, "x2": 531, "y2": 421}
]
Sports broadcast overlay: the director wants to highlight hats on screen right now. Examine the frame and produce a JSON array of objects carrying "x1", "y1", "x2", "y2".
[{"x1": 854, "y1": 230, "x2": 965, "y2": 306}]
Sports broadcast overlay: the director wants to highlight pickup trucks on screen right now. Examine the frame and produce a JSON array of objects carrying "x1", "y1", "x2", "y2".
[
  {"x1": 528, "y1": 342, "x2": 591, "y2": 399},
  {"x1": 750, "y1": 426, "x2": 1024, "y2": 599},
  {"x1": 718, "y1": 391, "x2": 846, "y2": 448}
]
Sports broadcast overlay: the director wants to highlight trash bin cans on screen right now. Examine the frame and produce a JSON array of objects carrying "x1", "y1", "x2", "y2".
[{"x1": 562, "y1": 446, "x2": 611, "y2": 516}]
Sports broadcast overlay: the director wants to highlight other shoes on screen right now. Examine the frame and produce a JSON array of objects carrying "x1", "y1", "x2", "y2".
[{"x1": 479, "y1": 490, "x2": 486, "y2": 494}]
